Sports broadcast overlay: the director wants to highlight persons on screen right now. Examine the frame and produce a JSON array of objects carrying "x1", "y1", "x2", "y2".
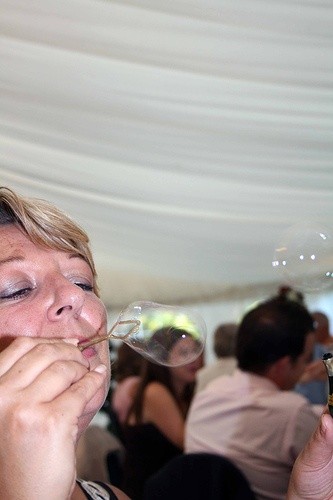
[
  {"x1": 118, "y1": 326, "x2": 204, "y2": 500},
  {"x1": 192, "y1": 322, "x2": 240, "y2": 398},
  {"x1": 0, "y1": 185, "x2": 333, "y2": 500},
  {"x1": 279, "y1": 284, "x2": 332, "y2": 415},
  {"x1": 111, "y1": 336, "x2": 149, "y2": 428},
  {"x1": 180, "y1": 296, "x2": 322, "y2": 499}
]
